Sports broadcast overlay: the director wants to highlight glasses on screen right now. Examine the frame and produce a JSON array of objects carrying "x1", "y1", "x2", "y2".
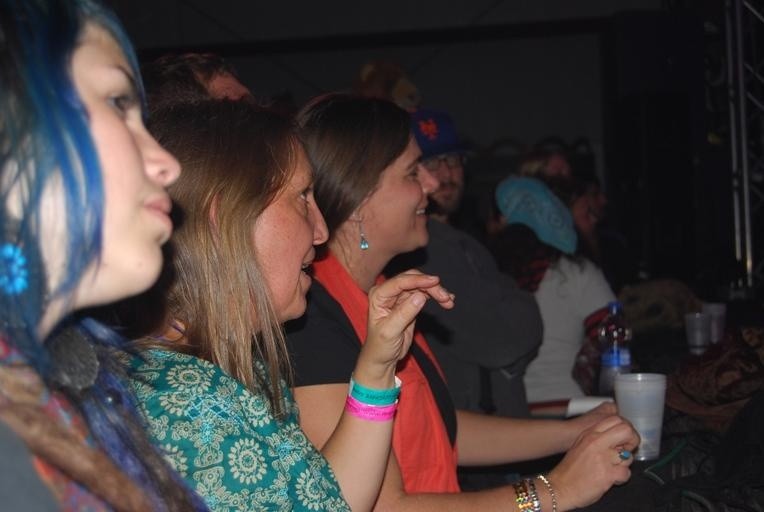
[{"x1": 424, "y1": 154, "x2": 460, "y2": 170}]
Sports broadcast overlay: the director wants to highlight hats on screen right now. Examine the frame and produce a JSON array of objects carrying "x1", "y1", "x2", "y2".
[{"x1": 407, "y1": 108, "x2": 457, "y2": 153}]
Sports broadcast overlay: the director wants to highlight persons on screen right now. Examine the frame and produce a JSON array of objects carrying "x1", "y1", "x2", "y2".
[{"x1": 0, "y1": 1, "x2": 763, "y2": 511}]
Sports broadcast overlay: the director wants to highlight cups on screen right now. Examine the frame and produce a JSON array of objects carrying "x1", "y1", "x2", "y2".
[
  {"x1": 703, "y1": 303, "x2": 727, "y2": 343},
  {"x1": 614, "y1": 374, "x2": 667, "y2": 461},
  {"x1": 685, "y1": 312, "x2": 710, "y2": 355}
]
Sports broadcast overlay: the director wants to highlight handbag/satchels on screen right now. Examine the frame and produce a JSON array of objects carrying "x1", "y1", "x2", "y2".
[{"x1": 675, "y1": 328, "x2": 763, "y2": 405}]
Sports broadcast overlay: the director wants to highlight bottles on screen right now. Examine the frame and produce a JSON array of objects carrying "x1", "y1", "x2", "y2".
[{"x1": 598, "y1": 301, "x2": 632, "y2": 393}]
